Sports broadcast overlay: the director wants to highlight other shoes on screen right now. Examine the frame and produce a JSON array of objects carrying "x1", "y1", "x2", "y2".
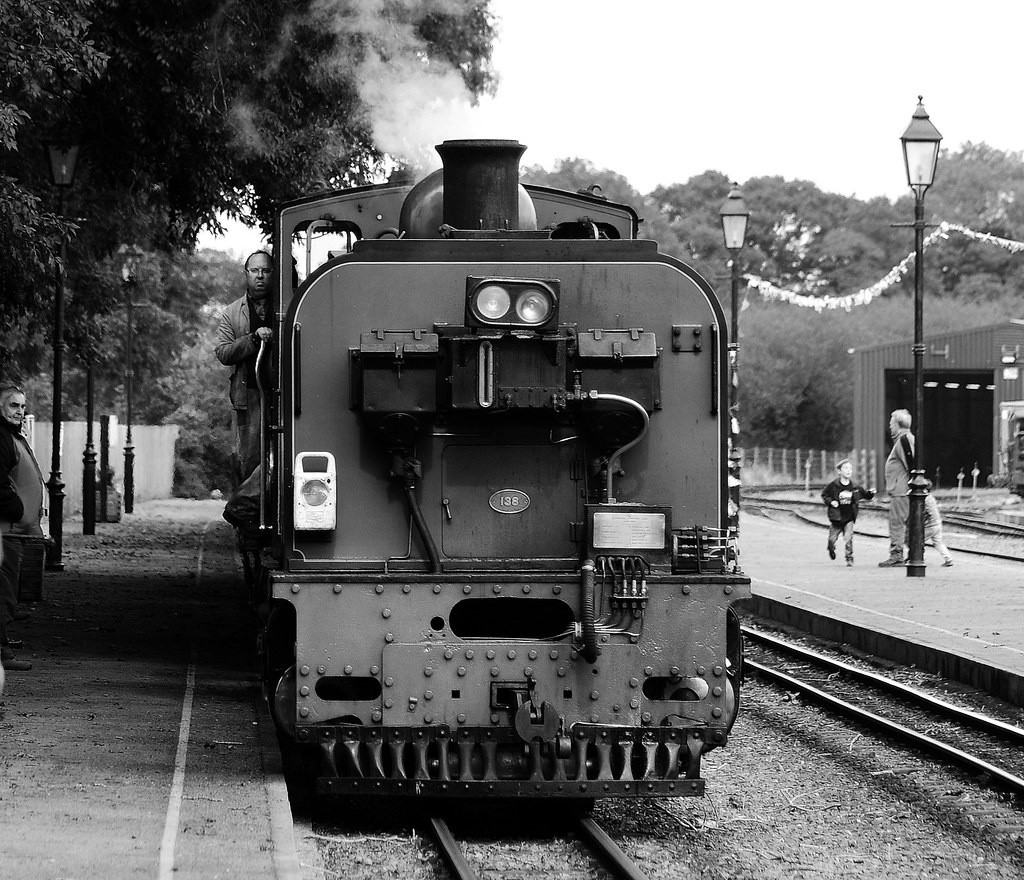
[
  {"x1": 847, "y1": 561, "x2": 853, "y2": 567},
  {"x1": 223, "y1": 510, "x2": 241, "y2": 528},
  {"x1": 830, "y1": 550, "x2": 836, "y2": 560},
  {"x1": 1, "y1": 658, "x2": 32, "y2": 671}
]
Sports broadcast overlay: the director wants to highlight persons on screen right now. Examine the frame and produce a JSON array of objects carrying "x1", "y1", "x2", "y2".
[
  {"x1": 0, "y1": 382, "x2": 44, "y2": 671},
  {"x1": 904, "y1": 479, "x2": 953, "y2": 566},
  {"x1": 879, "y1": 409, "x2": 914, "y2": 567},
  {"x1": 215, "y1": 250, "x2": 275, "y2": 535},
  {"x1": 821, "y1": 459, "x2": 877, "y2": 566}
]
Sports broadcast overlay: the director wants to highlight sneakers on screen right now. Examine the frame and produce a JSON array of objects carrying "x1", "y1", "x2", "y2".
[
  {"x1": 942, "y1": 561, "x2": 953, "y2": 567},
  {"x1": 878, "y1": 558, "x2": 904, "y2": 568},
  {"x1": 904, "y1": 558, "x2": 925, "y2": 565}
]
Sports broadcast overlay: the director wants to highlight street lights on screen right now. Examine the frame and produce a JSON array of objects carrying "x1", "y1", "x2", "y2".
[
  {"x1": 719, "y1": 181, "x2": 750, "y2": 544},
  {"x1": 118, "y1": 243, "x2": 144, "y2": 512},
  {"x1": 889, "y1": 92, "x2": 943, "y2": 580},
  {"x1": 719, "y1": 181, "x2": 749, "y2": 565},
  {"x1": 45, "y1": 142, "x2": 77, "y2": 576}
]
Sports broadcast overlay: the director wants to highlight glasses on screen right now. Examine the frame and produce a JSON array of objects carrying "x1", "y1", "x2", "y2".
[{"x1": 249, "y1": 268, "x2": 272, "y2": 273}]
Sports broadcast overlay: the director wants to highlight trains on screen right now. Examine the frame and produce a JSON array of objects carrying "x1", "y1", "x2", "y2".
[{"x1": 216, "y1": 139, "x2": 753, "y2": 814}]
